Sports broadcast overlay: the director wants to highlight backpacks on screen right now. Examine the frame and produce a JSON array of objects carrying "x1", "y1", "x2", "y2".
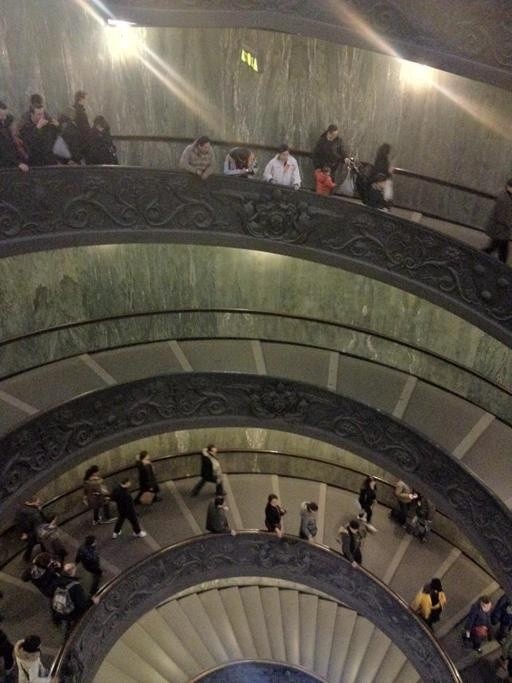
[{"x1": 53, "y1": 581, "x2": 80, "y2": 616}]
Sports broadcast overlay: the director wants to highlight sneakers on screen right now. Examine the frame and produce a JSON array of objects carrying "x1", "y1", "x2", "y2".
[
  {"x1": 134, "y1": 531, "x2": 146, "y2": 537},
  {"x1": 112, "y1": 531, "x2": 122, "y2": 538}
]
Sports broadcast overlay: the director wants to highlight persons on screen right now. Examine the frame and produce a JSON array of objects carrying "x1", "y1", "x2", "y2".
[
  {"x1": 408, "y1": 577, "x2": 446, "y2": 633},
  {"x1": 111, "y1": 476, "x2": 147, "y2": 538},
  {"x1": 369, "y1": 172, "x2": 385, "y2": 210},
  {"x1": 314, "y1": 167, "x2": 337, "y2": 195},
  {"x1": 0, "y1": 628, "x2": 16, "y2": 675},
  {"x1": 21, "y1": 553, "x2": 59, "y2": 599},
  {"x1": 13, "y1": 634, "x2": 61, "y2": 683},
  {"x1": 477, "y1": 178, "x2": 512, "y2": 264},
  {"x1": 265, "y1": 494, "x2": 288, "y2": 538},
  {"x1": 490, "y1": 594, "x2": 511, "y2": 647},
  {"x1": 32, "y1": 507, "x2": 69, "y2": 569},
  {"x1": 74, "y1": 536, "x2": 108, "y2": 598},
  {"x1": 189, "y1": 444, "x2": 227, "y2": 497},
  {"x1": 17, "y1": 496, "x2": 44, "y2": 552},
  {"x1": 462, "y1": 595, "x2": 493, "y2": 654},
  {"x1": 312, "y1": 124, "x2": 351, "y2": 196},
  {"x1": 133, "y1": 450, "x2": 164, "y2": 505},
  {"x1": 495, "y1": 630, "x2": 512, "y2": 683},
  {"x1": 179, "y1": 135, "x2": 217, "y2": 180},
  {"x1": 299, "y1": 501, "x2": 319, "y2": 543},
  {"x1": 337, "y1": 519, "x2": 361, "y2": 568},
  {"x1": 223, "y1": 145, "x2": 259, "y2": 179},
  {"x1": 264, "y1": 144, "x2": 302, "y2": 190},
  {"x1": 373, "y1": 141, "x2": 393, "y2": 174},
  {"x1": 358, "y1": 512, "x2": 368, "y2": 549},
  {"x1": 206, "y1": 495, "x2": 237, "y2": 538},
  {"x1": 0, "y1": 90, "x2": 119, "y2": 173},
  {"x1": 409, "y1": 494, "x2": 433, "y2": 544},
  {"x1": 394, "y1": 479, "x2": 417, "y2": 529},
  {"x1": 51, "y1": 561, "x2": 100, "y2": 639},
  {"x1": 358, "y1": 475, "x2": 377, "y2": 534},
  {"x1": 82, "y1": 464, "x2": 120, "y2": 525}
]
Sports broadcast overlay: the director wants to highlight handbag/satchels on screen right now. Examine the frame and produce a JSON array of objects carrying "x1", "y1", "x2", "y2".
[
  {"x1": 336, "y1": 526, "x2": 349, "y2": 543},
  {"x1": 472, "y1": 625, "x2": 487, "y2": 638},
  {"x1": 141, "y1": 492, "x2": 155, "y2": 505},
  {"x1": 339, "y1": 169, "x2": 354, "y2": 198},
  {"x1": 52, "y1": 136, "x2": 70, "y2": 159}
]
